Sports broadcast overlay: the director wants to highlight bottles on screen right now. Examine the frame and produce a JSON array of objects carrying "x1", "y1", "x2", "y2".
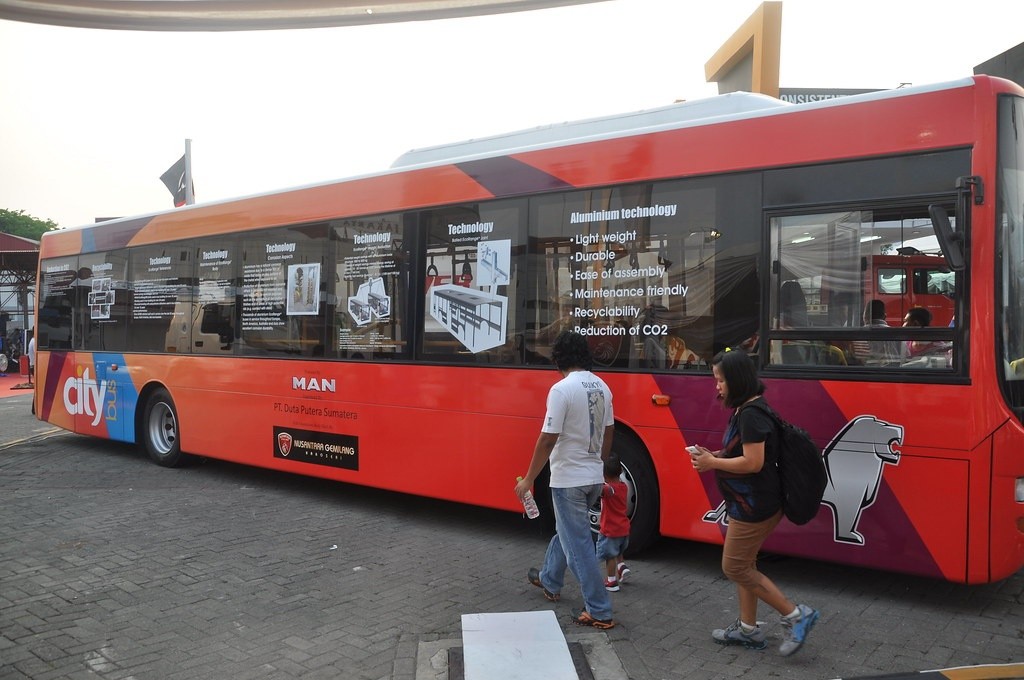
[{"x1": 516, "y1": 476, "x2": 540, "y2": 519}]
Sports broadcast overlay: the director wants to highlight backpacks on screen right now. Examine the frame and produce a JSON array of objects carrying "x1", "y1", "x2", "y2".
[{"x1": 729, "y1": 402, "x2": 829, "y2": 525}]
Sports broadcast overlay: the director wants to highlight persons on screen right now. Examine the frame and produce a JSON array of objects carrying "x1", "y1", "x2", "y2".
[
  {"x1": 900, "y1": 307, "x2": 944, "y2": 357},
  {"x1": 11, "y1": 328, "x2": 24, "y2": 346},
  {"x1": 514, "y1": 330, "x2": 618, "y2": 629},
  {"x1": 28, "y1": 327, "x2": 35, "y2": 416},
  {"x1": 690, "y1": 347, "x2": 818, "y2": 656},
  {"x1": 859, "y1": 299, "x2": 899, "y2": 362},
  {"x1": 602, "y1": 458, "x2": 633, "y2": 592}
]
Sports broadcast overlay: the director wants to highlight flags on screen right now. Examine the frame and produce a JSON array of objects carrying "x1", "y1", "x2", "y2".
[{"x1": 158, "y1": 155, "x2": 194, "y2": 207}]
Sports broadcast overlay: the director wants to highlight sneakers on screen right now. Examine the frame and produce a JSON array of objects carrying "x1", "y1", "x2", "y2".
[
  {"x1": 603, "y1": 578, "x2": 619, "y2": 591},
  {"x1": 712, "y1": 618, "x2": 768, "y2": 650},
  {"x1": 619, "y1": 565, "x2": 631, "y2": 583},
  {"x1": 569, "y1": 607, "x2": 615, "y2": 629},
  {"x1": 778, "y1": 604, "x2": 818, "y2": 656},
  {"x1": 528, "y1": 567, "x2": 560, "y2": 601}
]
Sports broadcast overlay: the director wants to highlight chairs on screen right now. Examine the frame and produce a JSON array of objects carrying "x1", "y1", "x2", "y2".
[
  {"x1": 782, "y1": 343, "x2": 852, "y2": 366},
  {"x1": 25, "y1": 354, "x2": 34, "y2": 383}
]
